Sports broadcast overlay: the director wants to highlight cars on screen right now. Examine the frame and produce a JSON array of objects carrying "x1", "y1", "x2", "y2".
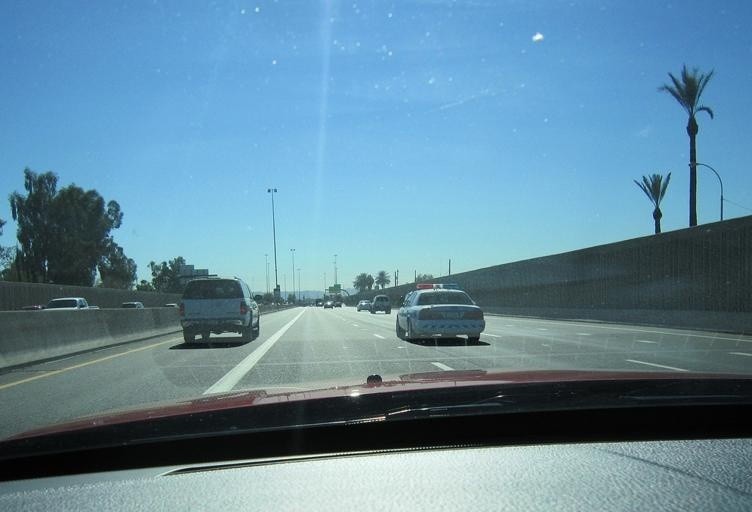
[
  {"x1": 22, "y1": 297, "x2": 178, "y2": 310},
  {"x1": 396, "y1": 283, "x2": 485, "y2": 346},
  {"x1": 315, "y1": 299, "x2": 372, "y2": 312}
]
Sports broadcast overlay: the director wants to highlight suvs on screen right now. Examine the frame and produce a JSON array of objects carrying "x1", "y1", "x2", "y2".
[
  {"x1": 370, "y1": 294, "x2": 391, "y2": 314},
  {"x1": 179, "y1": 276, "x2": 259, "y2": 344}
]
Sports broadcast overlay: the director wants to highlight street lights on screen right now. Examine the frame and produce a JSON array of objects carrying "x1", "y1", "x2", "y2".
[
  {"x1": 266, "y1": 188, "x2": 302, "y2": 306},
  {"x1": 687, "y1": 163, "x2": 723, "y2": 222}
]
